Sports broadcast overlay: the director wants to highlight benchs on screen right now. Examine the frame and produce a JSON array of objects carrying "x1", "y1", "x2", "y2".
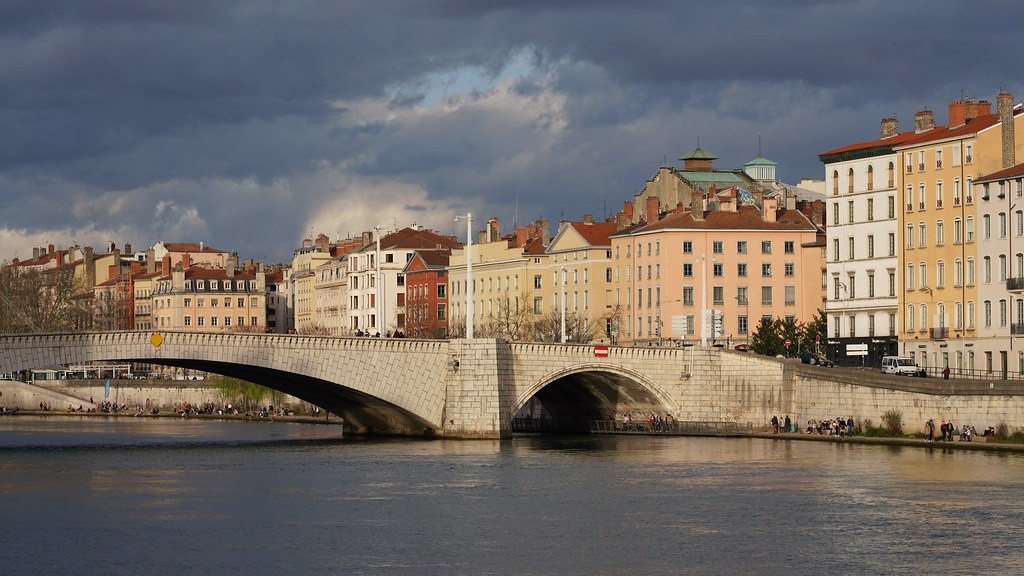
[
  {"x1": 798, "y1": 429, "x2": 831, "y2": 435},
  {"x1": 953, "y1": 435, "x2": 988, "y2": 443}
]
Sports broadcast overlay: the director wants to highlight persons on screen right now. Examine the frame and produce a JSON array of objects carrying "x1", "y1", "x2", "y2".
[
  {"x1": 649, "y1": 414, "x2": 674, "y2": 432},
  {"x1": 245, "y1": 410, "x2": 268, "y2": 417},
  {"x1": 68, "y1": 396, "x2": 129, "y2": 413},
  {"x1": 942, "y1": 366, "x2": 950, "y2": 380},
  {"x1": 225, "y1": 403, "x2": 238, "y2": 415},
  {"x1": 218, "y1": 409, "x2": 223, "y2": 415},
  {"x1": 925, "y1": 419, "x2": 995, "y2": 443},
  {"x1": 40, "y1": 402, "x2": 44, "y2": 411},
  {"x1": 622, "y1": 414, "x2": 629, "y2": 431},
  {"x1": 355, "y1": 329, "x2": 406, "y2": 338},
  {"x1": 286, "y1": 327, "x2": 298, "y2": 334},
  {"x1": 136, "y1": 398, "x2": 159, "y2": 415},
  {"x1": 173, "y1": 402, "x2": 214, "y2": 414},
  {"x1": 521, "y1": 413, "x2": 531, "y2": 426},
  {"x1": 265, "y1": 327, "x2": 273, "y2": 333},
  {"x1": 262, "y1": 405, "x2": 288, "y2": 416},
  {"x1": 312, "y1": 406, "x2": 319, "y2": 417},
  {"x1": 771, "y1": 415, "x2": 854, "y2": 437},
  {"x1": 919, "y1": 369, "x2": 927, "y2": 377}
]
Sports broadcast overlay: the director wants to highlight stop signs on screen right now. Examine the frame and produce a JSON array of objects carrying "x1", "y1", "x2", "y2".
[{"x1": 786, "y1": 339, "x2": 791, "y2": 346}]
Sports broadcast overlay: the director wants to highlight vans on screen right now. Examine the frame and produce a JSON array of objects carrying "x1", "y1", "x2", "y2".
[{"x1": 882, "y1": 356, "x2": 917, "y2": 375}]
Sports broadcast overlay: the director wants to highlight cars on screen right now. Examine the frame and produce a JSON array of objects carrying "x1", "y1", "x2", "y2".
[{"x1": 802, "y1": 353, "x2": 833, "y2": 368}]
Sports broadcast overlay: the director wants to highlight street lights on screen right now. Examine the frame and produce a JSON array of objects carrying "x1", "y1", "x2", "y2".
[
  {"x1": 454, "y1": 213, "x2": 479, "y2": 340},
  {"x1": 735, "y1": 295, "x2": 749, "y2": 352},
  {"x1": 374, "y1": 225, "x2": 387, "y2": 339}
]
